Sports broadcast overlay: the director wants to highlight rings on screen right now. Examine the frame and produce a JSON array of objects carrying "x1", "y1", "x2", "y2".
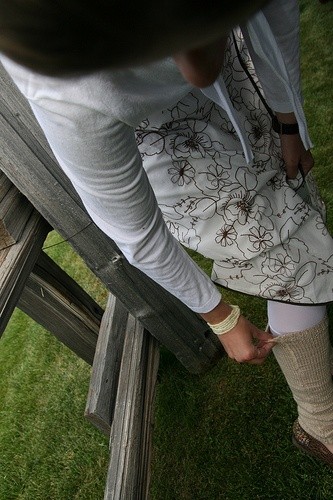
[{"x1": 256, "y1": 348, "x2": 262, "y2": 358}]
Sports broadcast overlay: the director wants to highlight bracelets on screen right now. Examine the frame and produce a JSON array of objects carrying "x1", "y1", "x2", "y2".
[
  {"x1": 207, "y1": 305, "x2": 240, "y2": 335},
  {"x1": 272, "y1": 116, "x2": 299, "y2": 135}
]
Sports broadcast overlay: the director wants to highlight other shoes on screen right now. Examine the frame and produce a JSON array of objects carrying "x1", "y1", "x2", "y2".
[{"x1": 292, "y1": 418, "x2": 333, "y2": 467}]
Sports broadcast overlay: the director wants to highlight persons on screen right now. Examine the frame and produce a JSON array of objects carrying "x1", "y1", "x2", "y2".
[{"x1": 0, "y1": 0, "x2": 333, "y2": 468}]
